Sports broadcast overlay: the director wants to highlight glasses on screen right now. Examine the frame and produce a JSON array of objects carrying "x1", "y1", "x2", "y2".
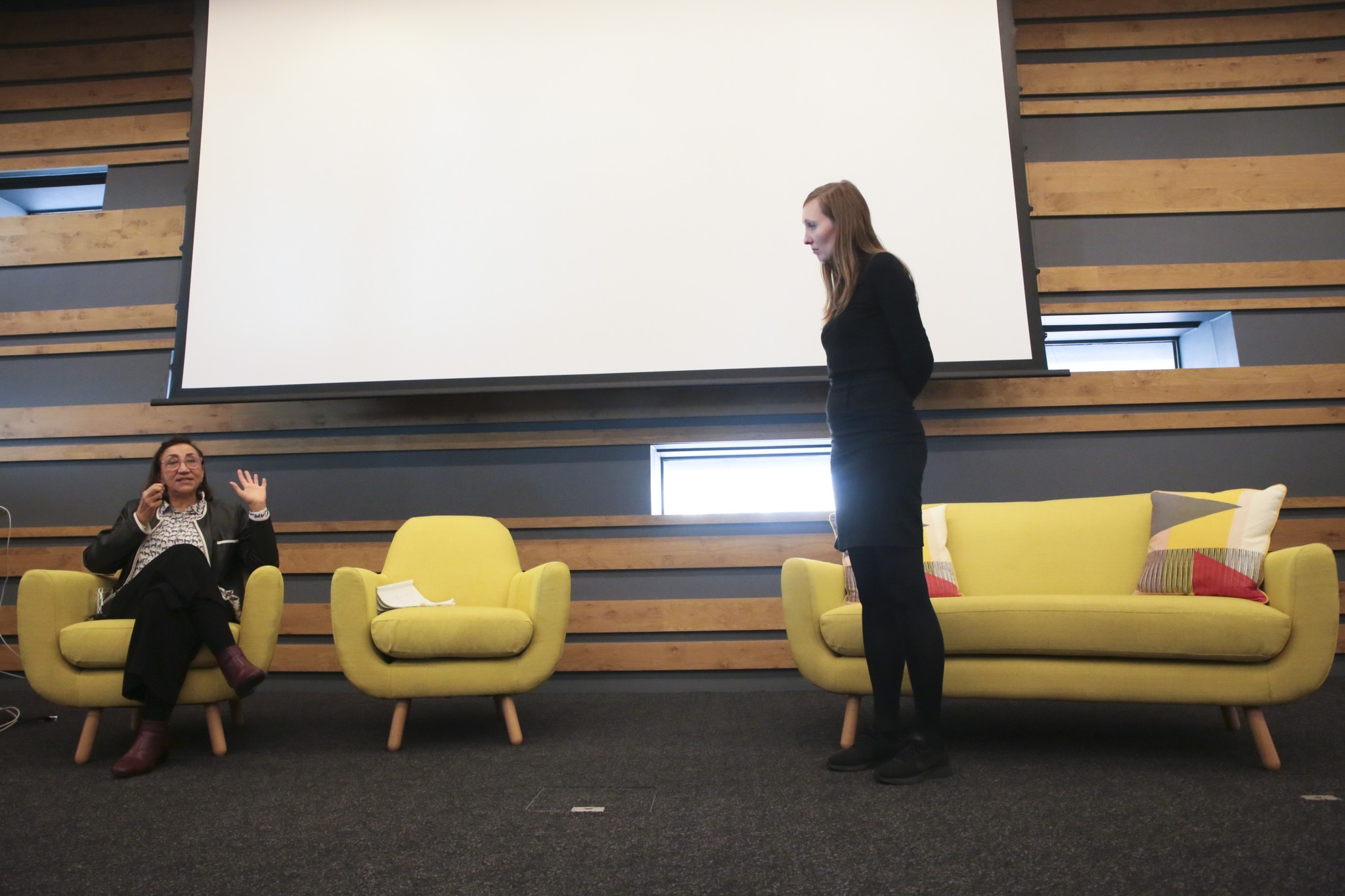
[{"x1": 159, "y1": 455, "x2": 202, "y2": 471}]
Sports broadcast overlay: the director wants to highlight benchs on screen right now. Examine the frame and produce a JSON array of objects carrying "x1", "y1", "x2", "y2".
[{"x1": 781, "y1": 484, "x2": 1340, "y2": 768}]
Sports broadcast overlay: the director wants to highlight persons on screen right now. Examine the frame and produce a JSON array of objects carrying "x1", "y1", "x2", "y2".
[
  {"x1": 803, "y1": 180, "x2": 952, "y2": 787},
  {"x1": 82, "y1": 436, "x2": 281, "y2": 776}
]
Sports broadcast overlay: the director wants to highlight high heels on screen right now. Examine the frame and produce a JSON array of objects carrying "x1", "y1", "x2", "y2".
[
  {"x1": 112, "y1": 721, "x2": 169, "y2": 776},
  {"x1": 216, "y1": 644, "x2": 266, "y2": 698}
]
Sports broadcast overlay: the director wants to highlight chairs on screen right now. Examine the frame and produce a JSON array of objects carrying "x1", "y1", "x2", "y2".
[
  {"x1": 328, "y1": 514, "x2": 572, "y2": 754},
  {"x1": 15, "y1": 564, "x2": 286, "y2": 765}
]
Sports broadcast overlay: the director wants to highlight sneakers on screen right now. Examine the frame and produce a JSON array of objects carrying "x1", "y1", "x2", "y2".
[
  {"x1": 874, "y1": 734, "x2": 958, "y2": 785},
  {"x1": 827, "y1": 727, "x2": 904, "y2": 773}
]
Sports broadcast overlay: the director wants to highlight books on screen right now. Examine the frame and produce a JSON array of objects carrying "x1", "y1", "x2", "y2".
[{"x1": 375, "y1": 579, "x2": 455, "y2": 613}]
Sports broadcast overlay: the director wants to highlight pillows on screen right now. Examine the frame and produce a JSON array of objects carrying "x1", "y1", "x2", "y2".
[
  {"x1": 1132, "y1": 485, "x2": 1288, "y2": 604},
  {"x1": 828, "y1": 502, "x2": 965, "y2": 607}
]
